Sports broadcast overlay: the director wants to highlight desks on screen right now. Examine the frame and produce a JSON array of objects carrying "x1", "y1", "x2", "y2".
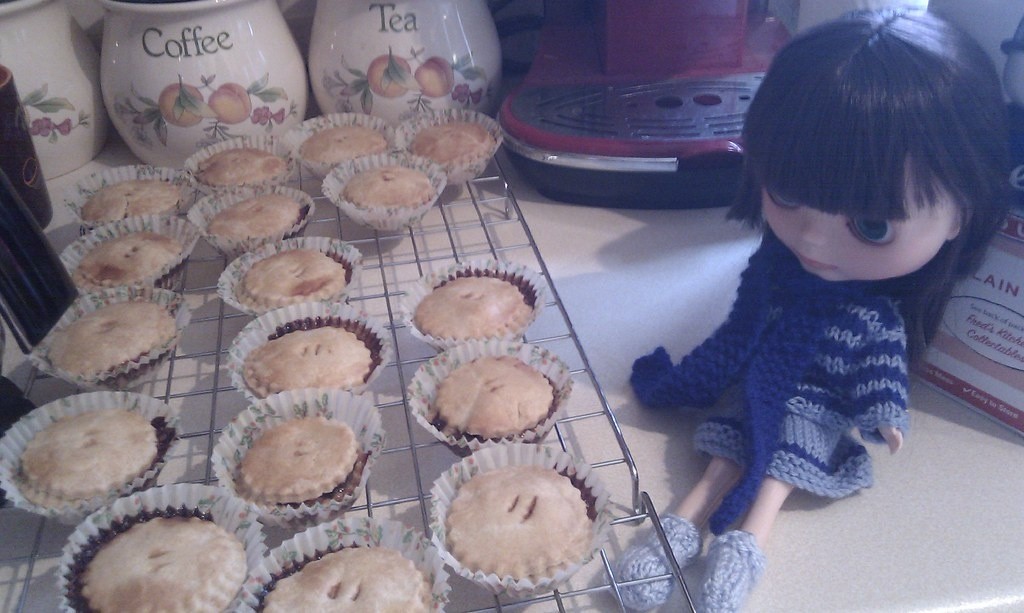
[{"x1": 2, "y1": 128, "x2": 1022, "y2": 611}]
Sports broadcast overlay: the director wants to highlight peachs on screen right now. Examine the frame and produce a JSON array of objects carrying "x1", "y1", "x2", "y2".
[
  {"x1": 157, "y1": 73, "x2": 252, "y2": 127},
  {"x1": 367, "y1": 45, "x2": 454, "y2": 98}
]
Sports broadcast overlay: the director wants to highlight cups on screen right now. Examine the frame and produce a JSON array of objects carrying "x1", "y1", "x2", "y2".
[
  {"x1": 0, "y1": 0, "x2": 512, "y2": 183},
  {"x1": 0, "y1": 63, "x2": 54, "y2": 233}
]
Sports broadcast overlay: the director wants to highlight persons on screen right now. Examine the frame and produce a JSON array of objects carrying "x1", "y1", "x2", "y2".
[{"x1": 604, "y1": 6, "x2": 1013, "y2": 612}]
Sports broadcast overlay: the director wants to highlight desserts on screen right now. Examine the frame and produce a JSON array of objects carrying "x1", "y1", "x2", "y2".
[{"x1": 0, "y1": 106, "x2": 613, "y2": 613}]
